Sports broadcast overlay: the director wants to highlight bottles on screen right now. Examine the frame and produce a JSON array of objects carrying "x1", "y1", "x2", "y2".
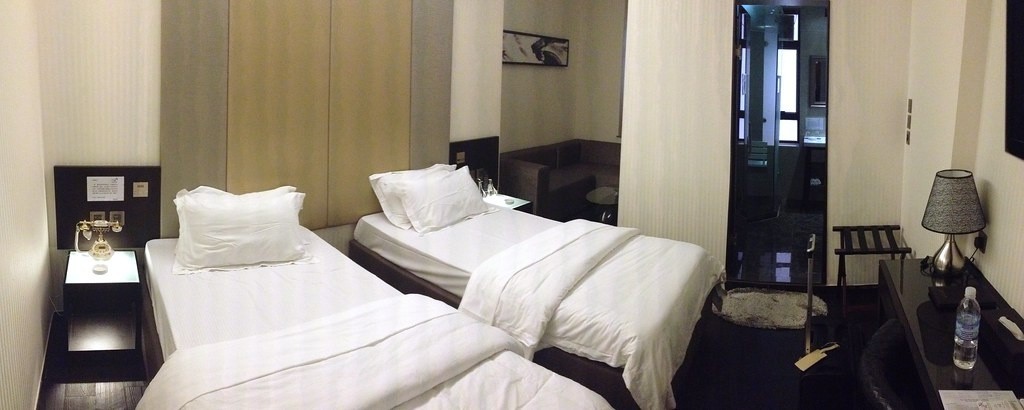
[
  {"x1": 478, "y1": 179, "x2": 498, "y2": 203},
  {"x1": 953, "y1": 286, "x2": 981, "y2": 371}
]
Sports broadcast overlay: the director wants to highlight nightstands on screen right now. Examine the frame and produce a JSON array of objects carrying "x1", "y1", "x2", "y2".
[
  {"x1": 63, "y1": 249, "x2": 141, "y2": 351},
  {"x1": 482, "y1": 194, "x2": 534, "y2": 214}
]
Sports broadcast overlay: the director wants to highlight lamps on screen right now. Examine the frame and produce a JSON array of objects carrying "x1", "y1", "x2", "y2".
[{"x1": 920, "y1": 169, "x2": 987, "y2": 277}]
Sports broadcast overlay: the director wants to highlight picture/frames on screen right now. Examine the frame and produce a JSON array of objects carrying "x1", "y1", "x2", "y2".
[{"x1": 502, "y1": 29, "x2": 570, "y2": 68}]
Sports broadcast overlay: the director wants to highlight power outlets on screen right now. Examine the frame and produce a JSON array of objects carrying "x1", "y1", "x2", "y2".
[
  {"x1": 89, "y1": 211, "x2": 105, "y2": 221},
  {"x1": 977, "y1": 230, "x2": 988, "y2": 253},
  {"x1": 456, "y1": 153, "x2": 466, "y2": 164},
  {"x1": 110, "y1": 211, "x2": 125, "y2": 226}
]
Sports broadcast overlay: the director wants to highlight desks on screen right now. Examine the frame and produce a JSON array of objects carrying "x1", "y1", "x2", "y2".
[
  {"x1": 585, "y1": 186, "x2": 619, "y2": 224},
  {"x1": 876, "y1": 260, "x2": 1024, "y2": 410}
]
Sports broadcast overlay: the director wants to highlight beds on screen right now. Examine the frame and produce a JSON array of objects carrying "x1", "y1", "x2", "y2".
[
  {"x1": 348, "y1": 211, "x2": 727, "y2": 410},
  {"x1": 135, "y1": 225, "x2": 618, "y2": 410}
]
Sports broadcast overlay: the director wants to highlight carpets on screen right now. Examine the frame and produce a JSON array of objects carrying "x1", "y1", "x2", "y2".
[
  {"x1": 712, "y1": 286, "x2": 829, "y2": 330},
  {"x1": 40, "y1": 310, "x2": 148, "y2": 385}
]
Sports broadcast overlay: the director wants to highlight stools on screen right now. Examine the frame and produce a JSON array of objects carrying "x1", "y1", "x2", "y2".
[{"x1": 831, "y1": 224, "x2": 912, "y2": 317}]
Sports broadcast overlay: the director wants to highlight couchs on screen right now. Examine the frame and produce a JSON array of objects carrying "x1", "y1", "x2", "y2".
[{"x1": 500, "y1": 137, "x2": 621, "y2": 222}]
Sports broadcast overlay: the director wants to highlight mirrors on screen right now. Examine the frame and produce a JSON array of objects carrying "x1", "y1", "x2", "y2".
[{"x1": 809, "y1": 55, "x2": 827, "y2": 108}]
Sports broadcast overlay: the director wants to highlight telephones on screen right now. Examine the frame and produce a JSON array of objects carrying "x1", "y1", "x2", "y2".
[{"x1": 75, "y1": 220, "x2": 122, "y2": 273}]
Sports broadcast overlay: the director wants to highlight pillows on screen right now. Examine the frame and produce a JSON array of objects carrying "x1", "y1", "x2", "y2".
[
  {"x1": 394, "y1": 166, "x2": 502, "y2": 236},
  {"x1": 368, "y1": 163, "x2": 457, "y2": 230},
  {"x1": 172, "y1": 184, "x2": 306, "y2": 276}
]
